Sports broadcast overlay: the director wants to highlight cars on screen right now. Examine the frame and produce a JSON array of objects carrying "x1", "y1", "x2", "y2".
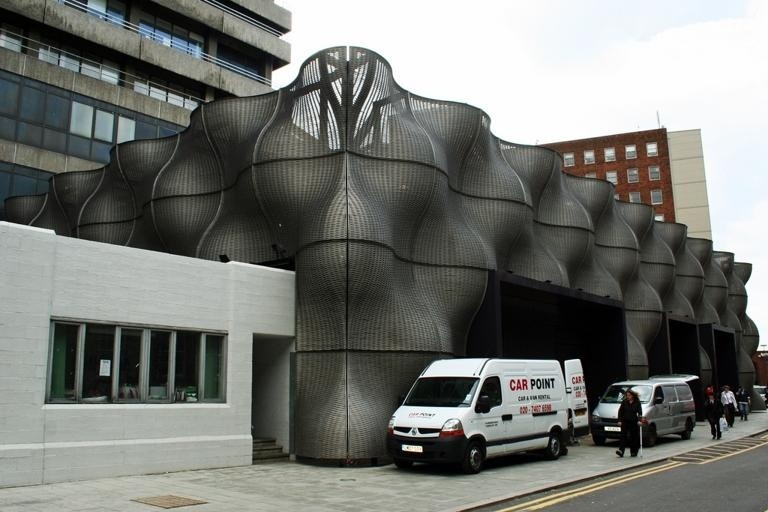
[{"x1": 746, "y1": 384, "x2": 767, "y2": 409}]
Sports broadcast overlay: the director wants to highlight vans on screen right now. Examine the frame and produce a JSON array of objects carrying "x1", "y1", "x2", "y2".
[
  {"x1": 382, "y1": 354, "x2": 589, "y2": 480},
  {"x1": 586, "y1": 369, "x2": 700, "y2": 449}
]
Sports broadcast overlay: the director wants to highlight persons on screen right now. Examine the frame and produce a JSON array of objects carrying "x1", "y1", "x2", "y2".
[
  {"x1": 703, "y1": 392, "x2": 726, "y2": 439},
  {"x1": 734, "y1": 384, "x2": 752, "y2": 421},
  {"x1": 615, "y1": 389, "x2": 642, "y2": 457},
  {"x1": 720, "y1": 384, "x2": 740, "y2": 428}
]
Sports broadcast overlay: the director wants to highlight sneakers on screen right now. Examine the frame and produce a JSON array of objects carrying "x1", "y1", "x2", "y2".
[{"x1": 615, "y1": 449, "x2": 638, "y2": 458}]
